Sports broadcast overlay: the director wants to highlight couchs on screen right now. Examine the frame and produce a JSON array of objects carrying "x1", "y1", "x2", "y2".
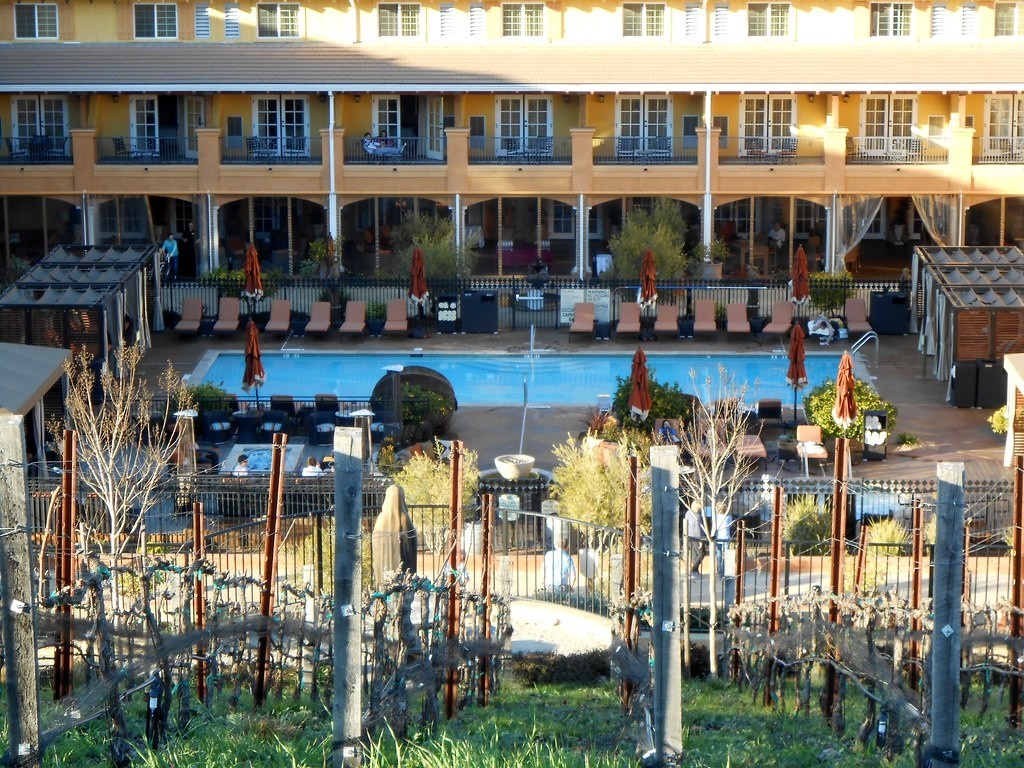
[{"x1": 203, "y1": 411, "x2": 394, "y2": 444}]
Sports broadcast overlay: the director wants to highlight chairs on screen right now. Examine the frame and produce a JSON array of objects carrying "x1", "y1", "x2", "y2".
[
  {"x1": 174, "y1": 298, "x2": 872, "y2": 344},
  {"x1": 506, "y1": 136, "x2": 553, "y2": 163},
  {"x1": 616, "y1": 136, "x2": 671, "y2": 165},
  {"x1": 167, "y1": 443, "x2": 219, "y2": 478},
  {"x1": 237, "y1": 394, "x2": 346, "y2": 417},
  {"x1": 846, "y1": 137, "x2": 923, "y2": 165},
  {"x1": 244, "y1": 135, "x2": 306, "y2": 163},
  {"x1": 111, "y1": 136, "x2": 137, "y2": 161},
  {"x1": 730, "y1": 494, "x2": 894, "y2": 547},
  {"x1": 746, "y1": 136, "x2": 797, "y2": 165},
  {"x1": 655, "y1": 399, "x2": 828, "y2": 472},
  {"x1": 5, "y1": 135, "x2": 68, "y2": 165}
]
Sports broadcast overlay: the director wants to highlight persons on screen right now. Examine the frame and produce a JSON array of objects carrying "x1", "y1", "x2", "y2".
[
  {"x1": 234, "y1": 455, "x2": 252, "y2": 477},
  {"x1": 162, "y1": 233, "x2": 179, "y2": 282},
  {"x1": 684, "y1": 499, "x2": 708, "y2": 577},
  {"x1": 360, "y1": 131, "x2": 406, "y2": 155},
  {"x1": 812, "y1": 314, "x2": 834, "y2": 346},
  {"x1": 372, "y1": 485, "x2": 418, "y2": 645},
  {"x1": 302, "y1": 454, "x2": 336, "y2": 478},
  {"x1": 181, "y1": 223, "x2": 195, "y2": 256},
  {"x1": 659, "y1": 420, "x2": 681, "y2": 445},
  {"x1": 714, "y1": 498, "x2": 733, "y2": 579},
  {"x1": 769, "y1": 221, "x2": 786, "y2": 260},
  {"x1": 899, "y1": 268, "x2": 913, "y2": 335}
]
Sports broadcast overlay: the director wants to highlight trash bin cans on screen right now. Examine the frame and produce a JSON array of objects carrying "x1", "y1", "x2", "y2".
[
  {"x1": 869, "y1": 291, "x2": 910, "y2": 335},
  {"x1": 437, "y1": 294, "x2": 457, "y2": 333},
  {"x1": 951, "y1": 361, "x2": 976, "y2": 408},
  {"x1": 863, "y1": 409, "x2": 889, "y2": 461},
  {"x1": 976, "y1": 358, "x2": 1002, "y2": 410},
  {"x1": 460, "y1": 290, "x2": 499, "y2": 335}
]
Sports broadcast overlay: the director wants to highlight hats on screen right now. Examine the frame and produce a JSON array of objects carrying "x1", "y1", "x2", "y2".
[{"x1": 238, "y1": 455, "x2": 248, "y2": 463}]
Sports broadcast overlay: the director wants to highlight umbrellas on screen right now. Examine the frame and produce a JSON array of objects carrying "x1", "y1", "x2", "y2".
[
  {"x1": 640, "y1": 246, "x2": 659, "y2": 309},
  {"x1": 790, "y1": 245, "x2": 811, "y2": 306},
  {"x1": 241, "y1": 320, "x2": 265, "y2": 394},
  {"x1": 241, "y1": 243, "x2": 264, "y2": 301},
  {"x1": 786, "y1": 320, "x2": 808, "y2": 390},
  {"x1": 832, "y1": 350, "x2": 857, "y2": 438},
  {"x1": 326, "y1": 234, "x2": 334, "y2": 266},
  {"x1": 408, "y1": 245, "x2": 430, "y2": 304}
]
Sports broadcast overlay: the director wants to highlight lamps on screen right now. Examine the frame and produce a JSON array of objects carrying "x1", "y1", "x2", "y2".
[
  {"x1": 319, "y1": 94, "x2": 326, "y2": 102},
  {"x1": 809, "y1": 94, "x2": 815, "y2": 103},
  {"x1": 112, "y1": 94, "x2": 120, "y2": 103},
  {"x1": 355, "y1": 94, "x2": 360, "y2": 102},
  {"x1": 843, "y1": 94, "x2": 850, "y2": 103},
  {"x1": 563, "y1": 96, "x2": 570, "y2": 103},
  {"x1": 598, "y1": 95, "x2": 605, "y2": 103}
]
